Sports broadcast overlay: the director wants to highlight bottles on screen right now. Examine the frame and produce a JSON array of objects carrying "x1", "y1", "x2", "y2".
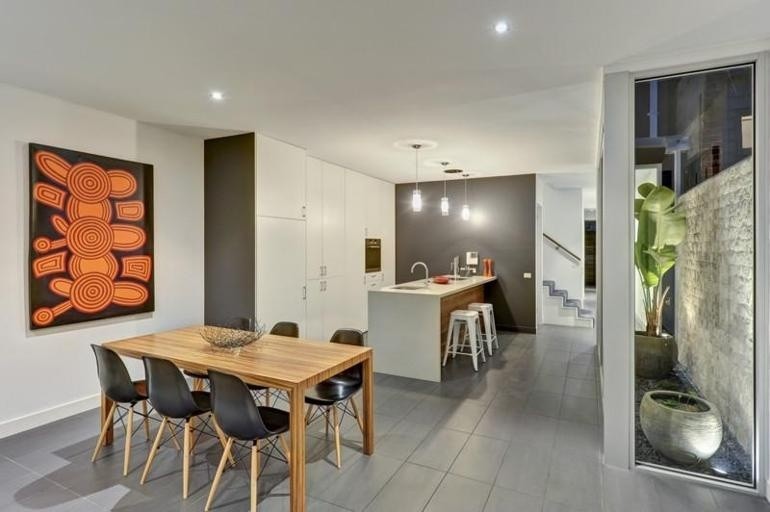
[{"x1": 450, "y1": 256, "x2": 493, "y2": 277}]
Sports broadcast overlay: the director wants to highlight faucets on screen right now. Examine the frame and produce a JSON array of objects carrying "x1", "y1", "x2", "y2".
[{"x1": 410, "y1": 261, "x2": 429, "y2": 281}]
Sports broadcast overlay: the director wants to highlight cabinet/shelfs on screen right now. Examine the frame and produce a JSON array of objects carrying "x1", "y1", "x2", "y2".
[
  {"x1": 204, "y1": 130, "x2": 307, "y2": 219},
  {"x1": 307, "y1": 156, "x2": 396, "y2": 344},
  {"x1": 204, "y1": 218, "x2": 306, "y2": 346}
]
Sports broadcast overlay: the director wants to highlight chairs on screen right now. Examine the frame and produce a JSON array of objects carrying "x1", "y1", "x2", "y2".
[{"x1": 89, "y1": 317, "x2": 375, "y2": 511}]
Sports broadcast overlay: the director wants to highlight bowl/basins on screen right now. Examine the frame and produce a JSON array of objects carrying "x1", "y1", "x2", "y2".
[{"x1": 434, "y1": 276, "x2": 449, "y2": 284}]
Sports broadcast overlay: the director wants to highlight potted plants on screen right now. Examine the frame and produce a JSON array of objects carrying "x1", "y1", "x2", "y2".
[{"x1": 634, "y1": 181, "x2": 690, "y2": 378}]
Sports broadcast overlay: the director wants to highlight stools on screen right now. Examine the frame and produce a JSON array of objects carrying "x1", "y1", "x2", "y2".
[
  {"x1": 461, "y1": 302, "x2": 499, "y2": 357},
  {"x1": 442, "y1": 309, "x2": 484, "y2": 374}
]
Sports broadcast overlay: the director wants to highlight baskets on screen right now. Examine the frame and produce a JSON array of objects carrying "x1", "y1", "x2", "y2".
[{"x1": 200, "y1": 323, "x2": 266, "y2": 346}]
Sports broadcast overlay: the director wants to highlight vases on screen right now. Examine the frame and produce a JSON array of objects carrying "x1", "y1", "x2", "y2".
[{"x1": 639, "y1": 390, "x2": 725, "y2": 465}]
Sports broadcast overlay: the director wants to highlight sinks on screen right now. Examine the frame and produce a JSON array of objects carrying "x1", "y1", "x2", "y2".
[{"x1": 388, "y1": 284, "x2": 424, "y2": 291}]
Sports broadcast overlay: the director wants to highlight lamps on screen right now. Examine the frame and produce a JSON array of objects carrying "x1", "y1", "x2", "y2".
[
  {"x1": 439, "y1": 161, "x2": 451, "y2": 217},
  {"x1": 410, "y1": 144, "x2": 423, "y2": 212},
  {"x1": 461, "y1": 174, "x2": 469, "y2": 220}
]
